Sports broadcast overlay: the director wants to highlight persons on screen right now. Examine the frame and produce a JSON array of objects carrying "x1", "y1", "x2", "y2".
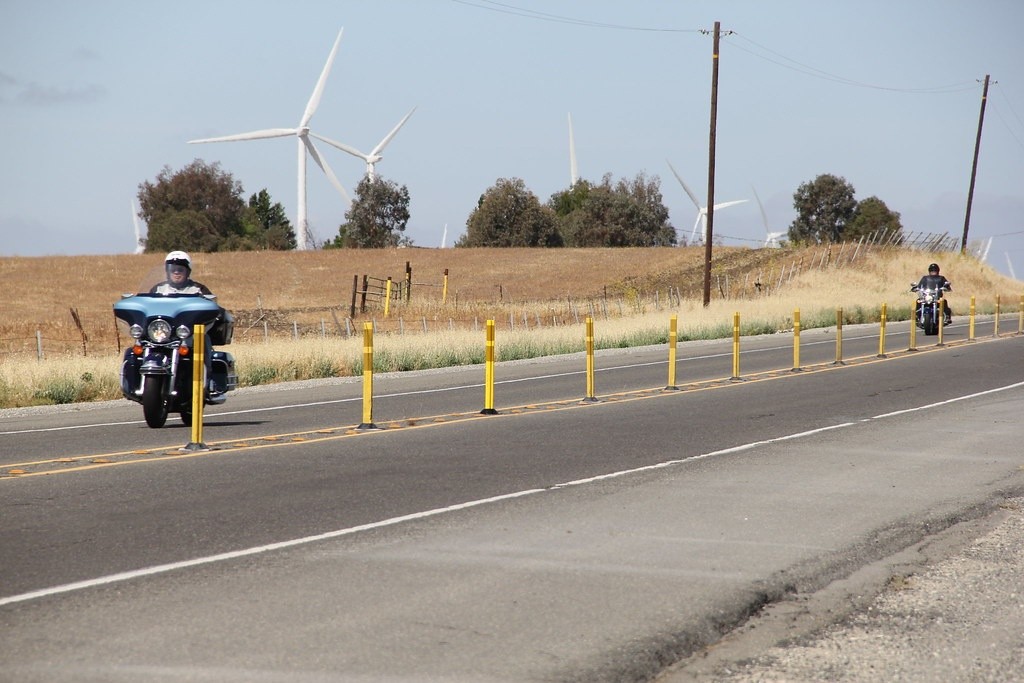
[
  {"x1": 910, "y1": 264, "x2": 952, "y2": 324},
  {"x1": 146, "y1": 251, "x2": 217, "y2": 397}
]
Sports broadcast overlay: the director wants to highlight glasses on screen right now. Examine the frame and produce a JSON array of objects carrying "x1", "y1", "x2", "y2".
[{"x1": 169, "y1": 267, "x2": 186, "y2": 272}]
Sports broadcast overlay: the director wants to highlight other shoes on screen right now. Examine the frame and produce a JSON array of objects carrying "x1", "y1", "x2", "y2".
[{"x1": 948, "y1": 319, "x2": 952, "y2": 324}]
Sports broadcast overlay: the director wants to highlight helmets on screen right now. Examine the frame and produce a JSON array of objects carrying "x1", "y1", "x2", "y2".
[
  {"x1": 164, "y1": 250, "x2": 192, "y2": 277},
  {"x1": 929, "y1": 264, "x2": 939, "y2": 272}
]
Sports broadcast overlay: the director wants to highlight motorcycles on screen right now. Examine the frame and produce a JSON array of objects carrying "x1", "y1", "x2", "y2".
[
  {"x1": 909, "y1": 273, "x2": 953, "y2": 336},
  {"x1": 111, "y1": 292, "x2": 240, "y2": 429}
]
